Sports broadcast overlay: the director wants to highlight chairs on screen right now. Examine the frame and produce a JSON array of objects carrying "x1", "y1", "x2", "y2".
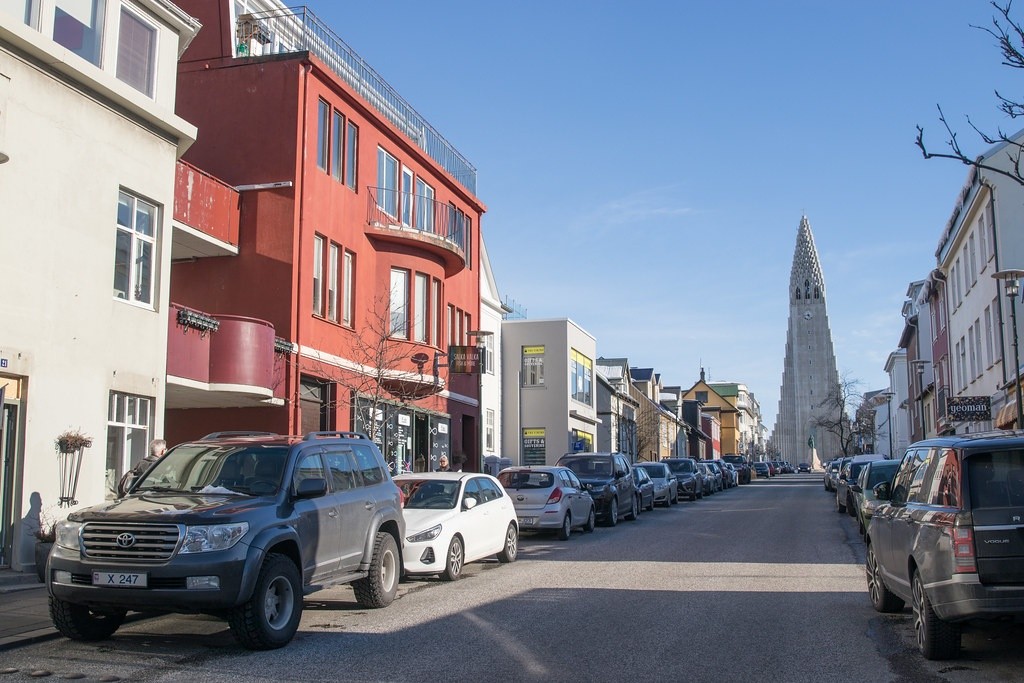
[
  {"x1": 217, "y1": 460, "x2": 238, "y2": 490},
  {"x1": 517, "y1": 473, "x2": 529, "y2": 487},
  {"x1": 593, "y1": 463, "x2": 605, "y2": 475}
]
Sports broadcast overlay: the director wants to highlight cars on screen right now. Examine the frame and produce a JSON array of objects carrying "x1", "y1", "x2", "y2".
[
  {"x1": 559, "y1": 450, "x2": 639, "y2": 528},
  {"x1": 44, "y1": 430, "x2": 405, "y2": 649},
  {"x1": 851, "y1": 460, "x2": 905, "y2": 540},
  {"x1": 824, "y1": 456, "x2": 886, "y2": 513},
  {"x1": 860, "y1": 437, "x2": 1023, "y2": 656},
  {"x1": 631, "y1": 455, "x2": 816, "y2": 513},
  {"x1": 388, "y1": 470, "x2": 518, "y2": 580},
  {"x1": 496, "y1": 465, "x2": 597, "y2": 539}
]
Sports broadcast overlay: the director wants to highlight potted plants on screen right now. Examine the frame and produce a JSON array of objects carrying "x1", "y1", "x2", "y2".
[
  {"x1": 55, "y1": 428, "x2": 92, "y2": 453},
  {"x1": 34, "y1": 525, "x2": 56, "y2": 582}
]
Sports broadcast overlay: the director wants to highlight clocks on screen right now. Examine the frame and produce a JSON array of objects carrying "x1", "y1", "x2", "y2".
[{"x1": 803, "y1": 310, "x2": 813, "y2": 320}]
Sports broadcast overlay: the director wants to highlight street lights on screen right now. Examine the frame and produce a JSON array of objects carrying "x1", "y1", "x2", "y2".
[
  {"x1": 882, "y1": 393, "x2": 897, "y2": 459},
  {"x1": 912, "y1": 359, "x2": 932, "y2": 441},
  {"x1": 465, "y1": 330, "x2": 495, "y2": 472},
  {"x1": 992, "y1": 267, "x2": 1024, "y2": 435}
]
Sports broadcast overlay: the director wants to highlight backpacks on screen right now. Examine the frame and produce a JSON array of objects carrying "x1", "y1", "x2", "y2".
[{"x1": 117, "y1": 467, "x2": 138, "y2": 499}]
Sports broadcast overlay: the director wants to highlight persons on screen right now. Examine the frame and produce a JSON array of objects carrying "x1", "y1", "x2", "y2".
[
  {"x1": 116, "y1": 439, "x2": 167, "y2": 501},
  {"x1": 435, "y1": 455, "x2": 453, "y2": 472}
]
